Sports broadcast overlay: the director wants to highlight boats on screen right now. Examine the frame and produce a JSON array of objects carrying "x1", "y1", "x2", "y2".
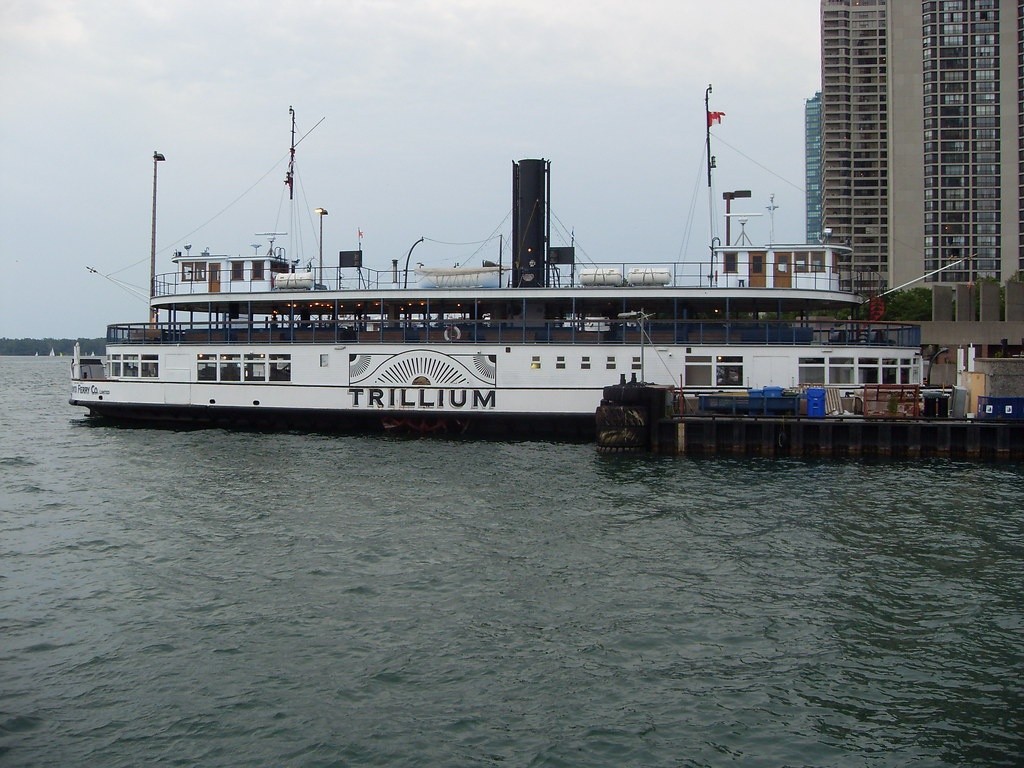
[
  {"x1": 413, "y1": 264, "x2": 512, "y2": 289},
  {"x1": 64, "y1": 82, "x2": 928, "y2": 441}
]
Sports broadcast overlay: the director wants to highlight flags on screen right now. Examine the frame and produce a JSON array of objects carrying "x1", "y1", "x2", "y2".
[{"x1": 708, "y1": 111, "x2": 726, "y2": 127}]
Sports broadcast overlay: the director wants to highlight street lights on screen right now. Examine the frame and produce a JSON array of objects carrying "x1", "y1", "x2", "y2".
[
  {"x1": 148, "y1": 148, "x2": 165, "y2": 330},
  {"x1": 314, "y1": 207, "x2": 328, "y2": 289},
  {"x1": 617, "y1": 308, "x2": 656, "y2": 383}
]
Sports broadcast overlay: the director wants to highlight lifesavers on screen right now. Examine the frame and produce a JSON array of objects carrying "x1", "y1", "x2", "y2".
[{"x1": 444, "y1": 326, "x2": 461, "y2": 342}]
